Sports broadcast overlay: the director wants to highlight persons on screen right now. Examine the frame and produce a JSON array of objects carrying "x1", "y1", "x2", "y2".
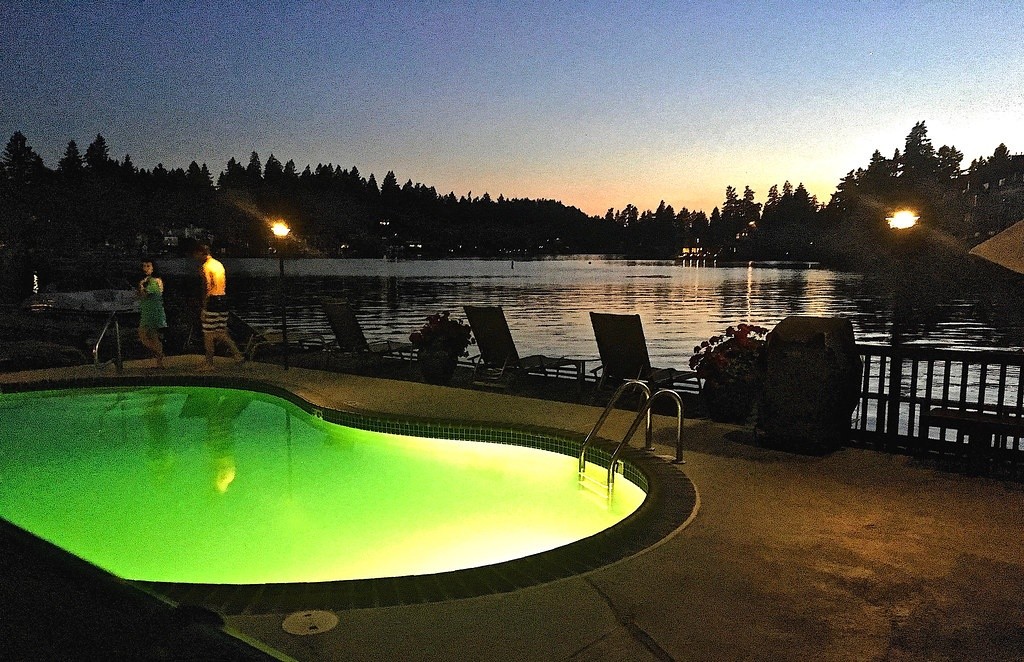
[
  {"x1": 136, "y1": 259, "x2": 170, "y2": 371},
  {"x1": 191, "y1": 244, "x2": 251, "y2": 373}
]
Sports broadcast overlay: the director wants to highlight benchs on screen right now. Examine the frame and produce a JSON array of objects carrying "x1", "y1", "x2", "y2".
[{"x1": 919, "y1": 408, "x2": 1024, "y2": 477}]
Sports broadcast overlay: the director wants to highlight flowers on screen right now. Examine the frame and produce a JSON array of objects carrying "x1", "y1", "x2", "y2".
[
  {"x1": 689, "y1": 324, "x2": 769, "y2": 389},
  {"x1": 409, "y1": 311, "x2": 471, "y2": 357}
]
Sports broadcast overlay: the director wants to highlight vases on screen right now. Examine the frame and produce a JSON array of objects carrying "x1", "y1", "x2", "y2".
[
  {"x1": 703, "y1": 381, "x2": 756, "y2": 424},
  {"x1": 417, "y1": 350, "x2": 458, "y2": 384}
]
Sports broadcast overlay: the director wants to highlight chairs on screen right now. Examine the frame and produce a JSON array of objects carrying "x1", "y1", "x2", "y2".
[
  {"x1": 462, "y1": 305, "x2": 583, "y2": 395},
  {"x1": 590, "y1": 312, "x2": 702, "y2": 413},
  {"x1": 321, "y1": 299, "x2": 417, "y2": 375}
]
